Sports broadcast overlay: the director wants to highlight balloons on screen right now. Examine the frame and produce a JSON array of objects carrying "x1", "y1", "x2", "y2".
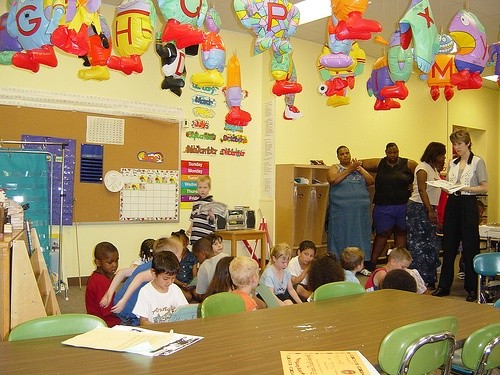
[{"x1": 0, "y1": 0, "x2": 500, "y2": 126}]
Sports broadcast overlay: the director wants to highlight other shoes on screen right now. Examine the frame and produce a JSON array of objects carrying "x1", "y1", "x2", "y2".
[
  {"x1": 360, "y1": 269, "x2": 371, "y2": 275},
  {"x1": 437, "y1": 264, "x2": 442, "y2": 273},
  {"x1": 366, "y1": 261, "x2": 377, "y2": 270},
  {"x1": 427, "y1": 282, "x2": 436, "y2": 290}
]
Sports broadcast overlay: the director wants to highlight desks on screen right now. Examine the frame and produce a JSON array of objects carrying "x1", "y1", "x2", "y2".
[
  {"x1": 0, "y1": 288, "x2": 500, "y2": 375},
  {"x1": 214, "y1": 229, "x2": 266, "y2": 272},
  {"x1": 0, "y1": 228, "x2": 28, "y2": 341}
]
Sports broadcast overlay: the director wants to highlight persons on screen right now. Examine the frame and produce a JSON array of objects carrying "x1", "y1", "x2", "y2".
[
  {"x1": 406, "y1": 142, "x2": 446, "y2": 290},
  {"x1": 431, "y1": 129, "x2": 488, "y2": 302},
  {"x1": 359, "y1": 143, "x2": 418, "y2": 272},
  {"x1": 187, "y1": 175, "x2": 217, "y2": 245},
  {"x1": 85, "y1": 229, "x2": 431, "y2": 326},
  {"x1": 456, "y1": 200, "x2": 488, "y2": 280},
  {"x1": 327, "y1": 146, "x2": 374, "y2": 276}
]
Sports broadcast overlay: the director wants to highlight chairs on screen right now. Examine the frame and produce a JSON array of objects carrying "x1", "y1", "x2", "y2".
[
  {"x1": 8, "y1": 314, "x2": 108, "y2": 342},
  {"x1": 313, "y1": 281, "x2": 364, "y2": 302},
  {"x1": 377, "y1": 252, "x2": 500, "y2": 375},
  {"x1": 201, "y1": 292, "x2": 246, "y2": 318}
]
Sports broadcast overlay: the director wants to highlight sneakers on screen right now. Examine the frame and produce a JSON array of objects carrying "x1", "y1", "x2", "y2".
[{"x1": 457, "y1": 272, "x2": 465, "y2": 279}]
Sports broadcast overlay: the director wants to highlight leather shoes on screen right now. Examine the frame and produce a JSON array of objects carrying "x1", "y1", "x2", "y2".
[
  {"x1": 431, "y1": 287, "x2": 450, "y2": 296},
  {"x1": 466, "y1": 294, "x2": 477, "y2": 301}
]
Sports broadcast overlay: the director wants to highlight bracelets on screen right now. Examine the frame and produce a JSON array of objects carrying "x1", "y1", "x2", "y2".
[{"x1": 468, "y1": 187, "x2": 471, "y2": 193}]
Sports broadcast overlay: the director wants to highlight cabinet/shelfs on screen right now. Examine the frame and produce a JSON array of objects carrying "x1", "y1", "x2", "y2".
[
  {"x1": 275, "y1": 164, "x2": 329, "y2": 249},
  {"x1": 11, "y1": 228, "x2": 61, "y2": 327}
]
